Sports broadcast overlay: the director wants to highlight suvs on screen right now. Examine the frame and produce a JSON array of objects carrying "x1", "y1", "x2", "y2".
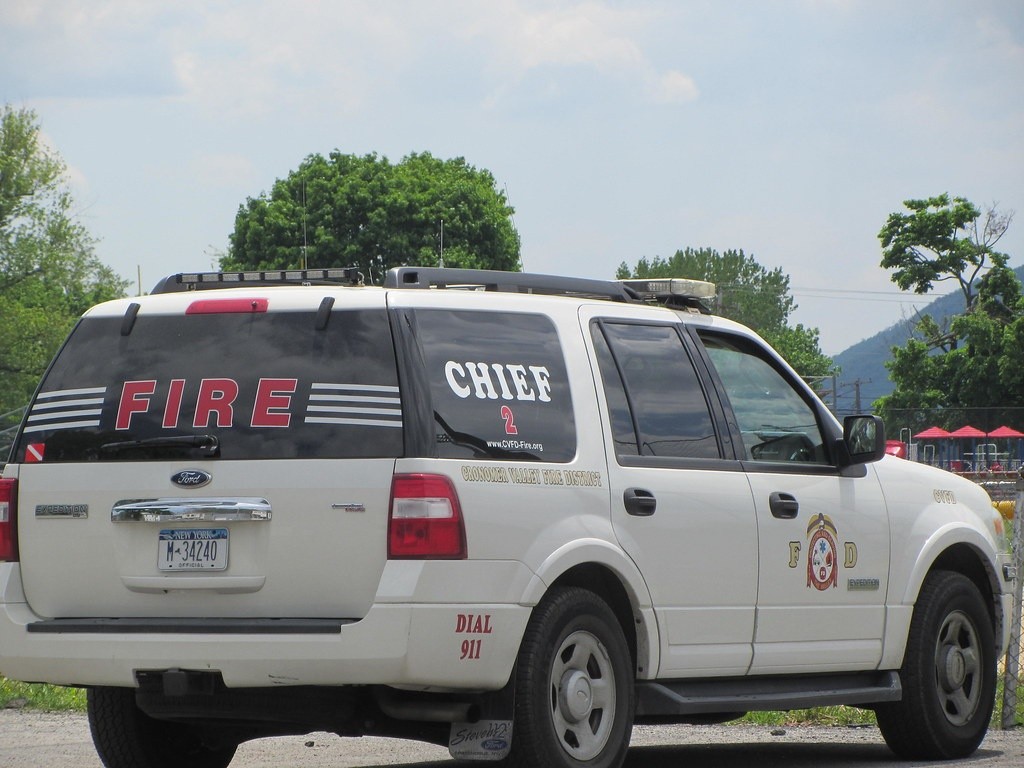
[{"x1": 1, "y1": 264, "x2": 1016, "y2": 768}]
